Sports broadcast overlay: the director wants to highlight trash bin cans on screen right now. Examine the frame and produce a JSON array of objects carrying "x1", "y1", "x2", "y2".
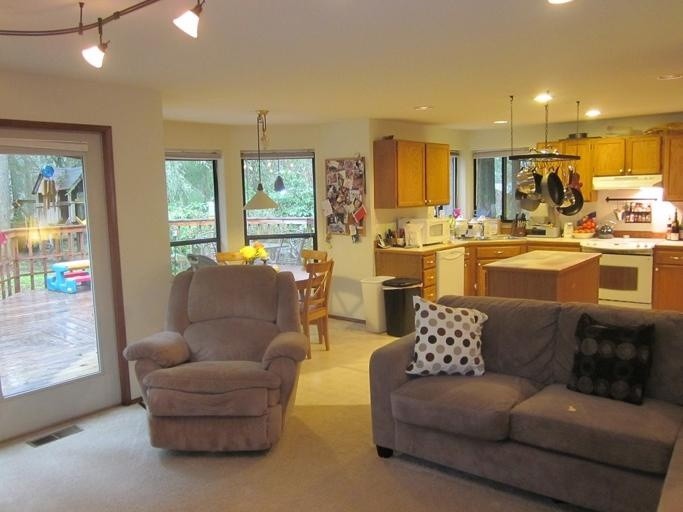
[{"x1": 360, "y1": 276, "x2": 423, "y2": 337}]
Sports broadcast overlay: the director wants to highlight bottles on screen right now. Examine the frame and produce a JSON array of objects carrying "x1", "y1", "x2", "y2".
[{"x1": 666, "y1": 208, "x2": 680, "y2": 241}]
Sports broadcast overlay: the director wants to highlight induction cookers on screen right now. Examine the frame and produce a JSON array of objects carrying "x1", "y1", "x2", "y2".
[{"x1": 580, "y1": 237, "x2": 656, "y2": 250}]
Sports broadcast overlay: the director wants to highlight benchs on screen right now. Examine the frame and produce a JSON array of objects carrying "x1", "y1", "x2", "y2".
[
  {"x1": 47, "y1": 271, "x2": 89, "y2": 291},
  {"x1": 64, "y1": 275, "x2": 91, "y2": 294}
]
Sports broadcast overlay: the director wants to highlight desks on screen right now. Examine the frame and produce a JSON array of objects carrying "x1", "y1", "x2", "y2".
[
  {"x1": 250, "y1": 242, "x2": 290, "y2": 262},
  {"x1": 51, "y1": 260, "x2": 90, "y2": 293}
]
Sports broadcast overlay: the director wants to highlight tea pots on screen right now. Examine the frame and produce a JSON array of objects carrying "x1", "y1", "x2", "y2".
[{"x1": 598, "y1": 223, "x2": 612, "y2": 238}]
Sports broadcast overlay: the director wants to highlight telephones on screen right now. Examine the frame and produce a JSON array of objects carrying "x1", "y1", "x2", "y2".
[{"x1": 377, "y1": 234, "x2": 390, "y2": 249}]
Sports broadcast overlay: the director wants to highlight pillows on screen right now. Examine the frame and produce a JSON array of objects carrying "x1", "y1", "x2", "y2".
[
  {"x1": 405, "y1": 295, "x2": 488, "y2": 377},
  {"x1": 552, "y1": 302, "x2": 683, "y2": 407},
  {"x1": 567, "y1": 313, "x2": 658, "y2": 406},
  {"x1": 437, "y1": 295, "x2": 562, "y2": 385}
]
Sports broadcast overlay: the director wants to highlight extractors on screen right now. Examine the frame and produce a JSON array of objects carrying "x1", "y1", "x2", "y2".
[{"x1": 591, "y1": 174, "x2": 663, "y2": 191}]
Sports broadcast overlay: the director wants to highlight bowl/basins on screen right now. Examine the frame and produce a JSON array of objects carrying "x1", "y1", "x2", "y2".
[
  {"x1": 568, "y1": 133, "x2": 587, "y2": 140},
  {"x1": 572, "y1": 233, "x2": 594, "y2": 239}
]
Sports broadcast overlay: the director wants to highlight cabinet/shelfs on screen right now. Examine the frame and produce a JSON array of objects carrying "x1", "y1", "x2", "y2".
[
  {"x1": 373, "y1": 139, "x2": 450, "y2": 209},
  {"x1": 653, "y1": 244, "x2": 683, "y2": 313},
  {"x1": 536, "y1": 131, "x2": 683, "y2": 202},
  {"x1": 375, "y1": 243, "x2": 581, "y2": 303}
]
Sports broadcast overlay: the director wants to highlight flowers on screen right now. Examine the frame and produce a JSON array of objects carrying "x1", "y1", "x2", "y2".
[{"x1": 240, "y1": 240, "x2": 268, "y2": 264}]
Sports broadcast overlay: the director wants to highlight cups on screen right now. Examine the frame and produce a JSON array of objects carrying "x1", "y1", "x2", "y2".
[{"x1": 396, "y1": 238, "x2": 404, "y2": 246}]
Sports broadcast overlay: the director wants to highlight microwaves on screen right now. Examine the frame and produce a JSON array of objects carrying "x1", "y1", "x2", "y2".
[{"x1": 399, "y1": 217, "x2": 451, "y2": 243}]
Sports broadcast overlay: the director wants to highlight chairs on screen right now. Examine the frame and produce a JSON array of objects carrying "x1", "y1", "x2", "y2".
[
  {"x1": 270, "y1": 229, "x2": 305, "y2": 264},
  {"x1": 299, "y1": 249, "x2": 327, "y2": 267},
  {"x1": 298, "y1": 260, "x2": 335, "y2": 359},
  {"x1": 123, "y1": 264, "x2": 309, "y2": 453},
  {"x1": 196, "y1": 231, "x2": 217, "y2": 263},
  {"x1": 215, "y1": 251, "x2": 249, "y2": 265},
  {"x1": 187, "y1": 253, "x2": 218, "y2": 271}
]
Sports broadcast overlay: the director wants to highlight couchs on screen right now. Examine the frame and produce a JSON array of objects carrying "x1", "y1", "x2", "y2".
[{"x1": 370, "y1": 295, "x2": 683, "y2": 512}]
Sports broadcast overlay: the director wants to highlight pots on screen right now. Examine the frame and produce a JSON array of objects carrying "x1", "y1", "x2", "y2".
[{"x1": 514, "y1": 160, "x2": 584, "y2": 217}]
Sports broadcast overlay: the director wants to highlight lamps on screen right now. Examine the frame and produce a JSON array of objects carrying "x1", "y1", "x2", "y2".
[
  {"x1": 242, "y1": 110, "x2": 279, "y2": 210},
  {"x1": 274, "y1": 160, "x2": 285, "y2": 192},
  {"x1": 0, "y1": 0, "x2": 205, "y2": 68}
]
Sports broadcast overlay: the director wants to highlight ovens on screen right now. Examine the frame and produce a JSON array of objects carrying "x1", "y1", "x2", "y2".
[{"x1": 581, "y1": 253, "x2": 653, "y2": 309}]
